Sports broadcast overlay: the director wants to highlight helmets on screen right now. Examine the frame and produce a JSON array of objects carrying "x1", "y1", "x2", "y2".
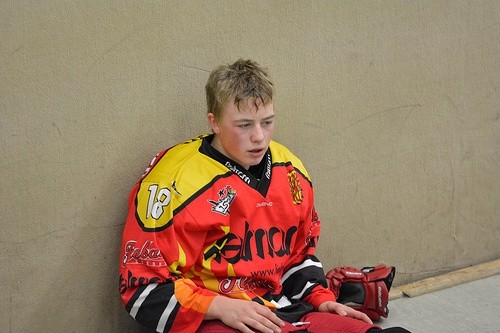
[{"x1": 324, "y1": 265, "x2": 395, "y2": 322}]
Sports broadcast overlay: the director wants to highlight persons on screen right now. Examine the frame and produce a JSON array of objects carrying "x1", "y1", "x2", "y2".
[{"x1": 119, "y1": 57, "x2": 412, "y2": 333}]
